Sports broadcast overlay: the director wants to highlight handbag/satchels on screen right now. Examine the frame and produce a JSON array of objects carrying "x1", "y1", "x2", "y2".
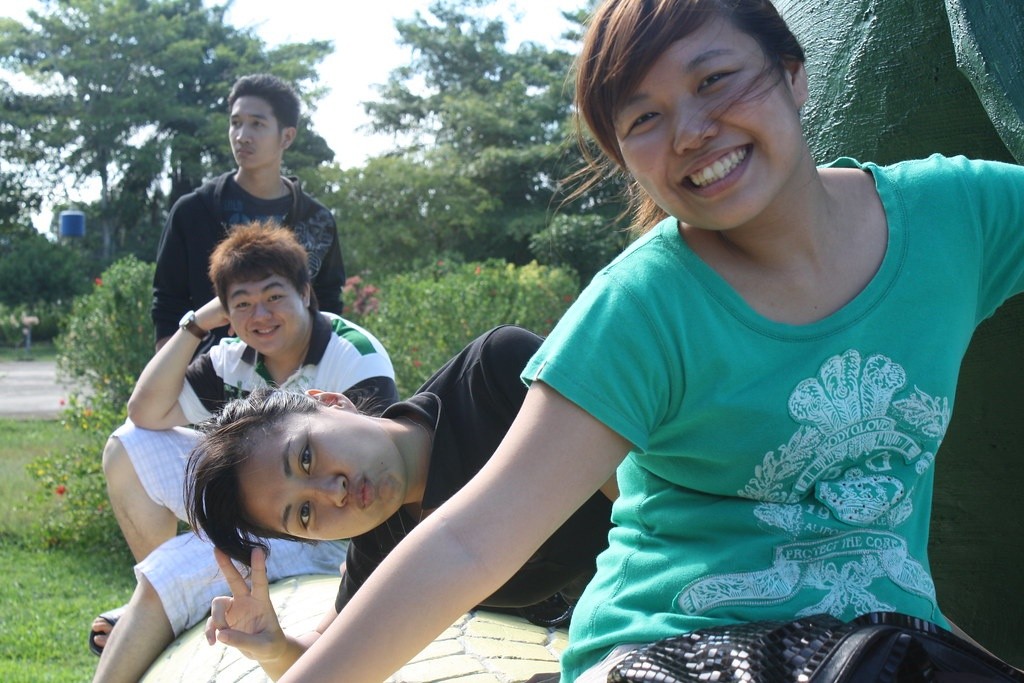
[{"x1": 607, "y1": 612, "x2": 1024, "y2": 683}]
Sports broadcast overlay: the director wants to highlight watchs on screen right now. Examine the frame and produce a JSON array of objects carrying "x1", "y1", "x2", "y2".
[{"x1": 179, "y1": 310, "x2": 211, "y2": 341}]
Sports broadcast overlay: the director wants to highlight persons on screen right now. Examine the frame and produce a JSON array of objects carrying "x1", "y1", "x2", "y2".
[
  {"x1": 149, "y1": 73, "x2": 350, "y2": 354},
  {"x1": 181, "y1": 322, "x2": 620, "y2": 683},
  {"x1": 273, "y1": 0, "x2": 1024, "y2": 683},
  {"x1": 91, "y1": 217, "x2": 401, "y2": 682}
]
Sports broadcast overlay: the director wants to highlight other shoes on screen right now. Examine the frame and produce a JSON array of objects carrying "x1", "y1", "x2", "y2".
[{"x1": 89, "y1": 604, "x2": 130, "y2": 657}]
